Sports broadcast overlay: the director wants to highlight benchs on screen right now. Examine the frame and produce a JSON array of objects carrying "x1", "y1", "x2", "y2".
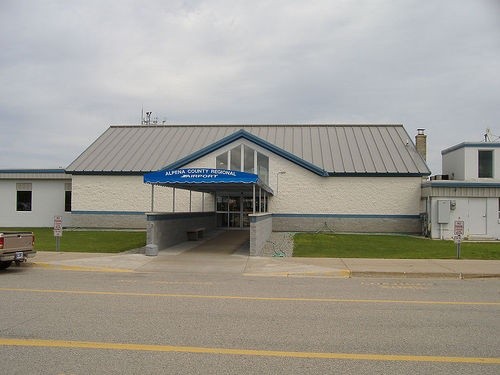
[{"x1": 186, "y1": 227, "x2": 206, "y2": 240}]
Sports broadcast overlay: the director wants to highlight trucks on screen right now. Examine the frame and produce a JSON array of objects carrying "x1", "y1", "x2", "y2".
[{"x1": 0, "y1": 230, "x2": 36, "y2": 268}]
